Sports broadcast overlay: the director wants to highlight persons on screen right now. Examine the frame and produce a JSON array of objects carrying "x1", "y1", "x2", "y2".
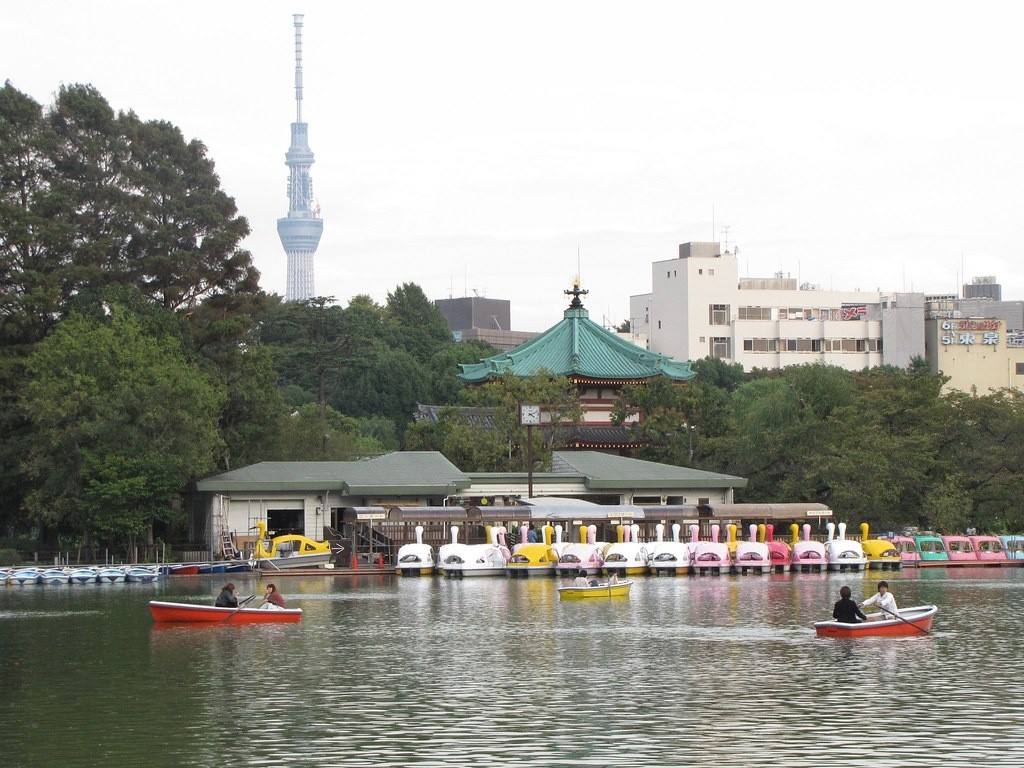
[
  {"x1": 259, "y1": 584, "x2": 286, "y2": 609},
  {"x1": 215, "y1": 583, "x2": 247, "y2": 608},
  {"x1": 573, "y1": 569, "x2": 589, "y2": 587},
  {"x1": 528, "y1": 524, "x2": 537, "y2": 543},
  {"x1": 832, "y1": 586, "x2": 868, "y2": 624},
  {"x1": 857, "y1": 581, "x2": 900, "y2": 622}
]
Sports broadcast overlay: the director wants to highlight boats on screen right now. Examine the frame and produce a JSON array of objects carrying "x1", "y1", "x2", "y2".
[
  {"x1": 877, "y1": 536, "x2": 1024, "y2": 568},
  {"x1": 125, "y1": 568, "x2": 158, "y2": 582},
  {"x1": 97, "y1": 569, "x2": 127, "y2": 583},
  {"x1": 9, "y1": 571, "x2": 41, "y2": 585},
  {"x1": 395, "y1": 526, "x2": 435, "y2": 577},
  {"x1": 558, "y1": 582, "x2": 634, "y2": 599},
  {"x1": 69, "y1": 570, "x2": 97, "y2": 583},
  {"x1": 249, "y1": 521, "x2": 333, "y2": 570},
  {"x1": 149, "y1": 600, "x2": 303, "y2": 623},
  {"x1": 436, "y1": 523, "x2": 903, "y2": 578},
  {"x1": 0, "y1": 564, "x2": 250, "y2": 574},
  {"x1": 40, "y1": 570, "x2": 70, "y2": 584},
  {"x1": 813, "y1": 605, "x2": 938, "y2": 638},
  {"x1": 0, "y1": 571, "x2": 9, "y2": 585}
]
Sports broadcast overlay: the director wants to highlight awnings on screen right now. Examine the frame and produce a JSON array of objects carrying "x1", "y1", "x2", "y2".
[{"x1": 516, "y1": 496, "x2": 600, "y2": 506}]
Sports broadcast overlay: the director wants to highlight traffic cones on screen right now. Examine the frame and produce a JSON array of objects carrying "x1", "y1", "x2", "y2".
[
  {"x1": 378, "y1": 552, "x2": 384, "y2": 569},
  {"x1": 352, "y1": 553, "x2": 359, "y2": 569}
]
